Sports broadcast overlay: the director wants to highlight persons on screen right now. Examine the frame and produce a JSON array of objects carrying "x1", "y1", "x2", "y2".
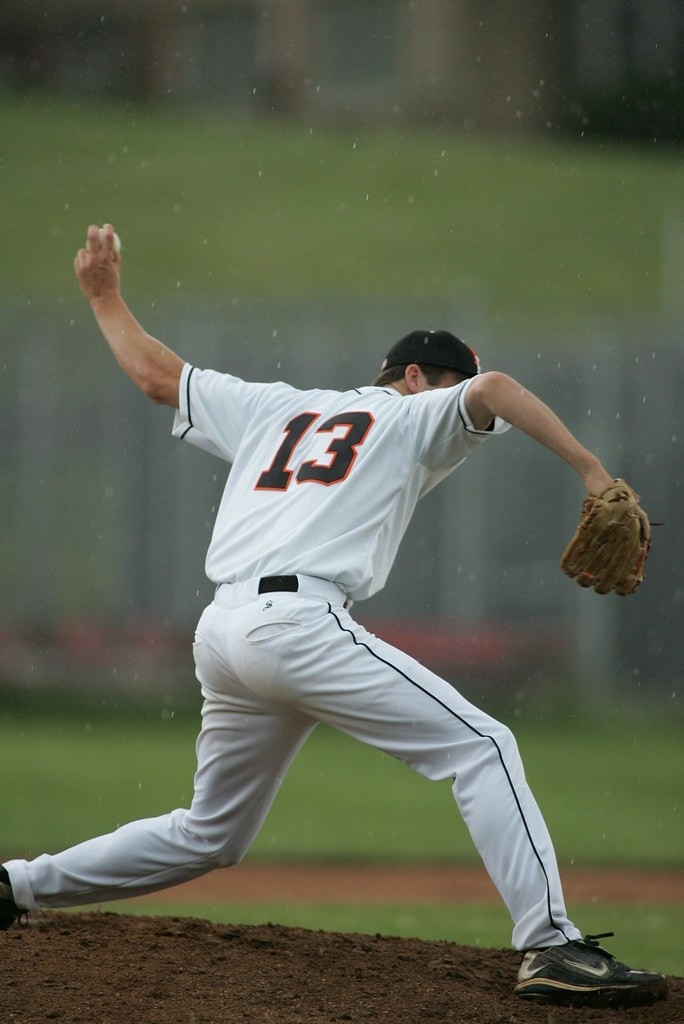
[{"x1": 0, "y1": 223, "x2": 668, "y2": 1007}]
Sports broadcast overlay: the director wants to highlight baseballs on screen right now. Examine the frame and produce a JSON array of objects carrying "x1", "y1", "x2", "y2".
[{"x1": 85, "y1": 229, "x2": 120, "y2": 262}]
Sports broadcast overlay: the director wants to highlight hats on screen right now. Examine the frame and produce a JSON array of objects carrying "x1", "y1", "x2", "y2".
[{"x1": 382, "y1": 327, "x2": 479, "y2": 375}]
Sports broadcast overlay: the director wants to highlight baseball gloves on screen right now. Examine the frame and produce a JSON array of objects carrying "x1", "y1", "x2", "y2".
[{"x1": 558, "y1": 475, "x2": 663, "y2": 597}]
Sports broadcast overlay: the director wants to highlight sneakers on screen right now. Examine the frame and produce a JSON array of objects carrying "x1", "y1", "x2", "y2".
[
  {"x1": 0, "y1": 861, "x2": 31, "y2": 931},
  {"x1": 513, "y1": 931, "x2": 670, "y2": 1007}
]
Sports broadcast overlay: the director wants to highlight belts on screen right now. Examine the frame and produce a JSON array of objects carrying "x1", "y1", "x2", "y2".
[{"x1": 257, "y1": 573, "x2": 299, "y2": 595}]
